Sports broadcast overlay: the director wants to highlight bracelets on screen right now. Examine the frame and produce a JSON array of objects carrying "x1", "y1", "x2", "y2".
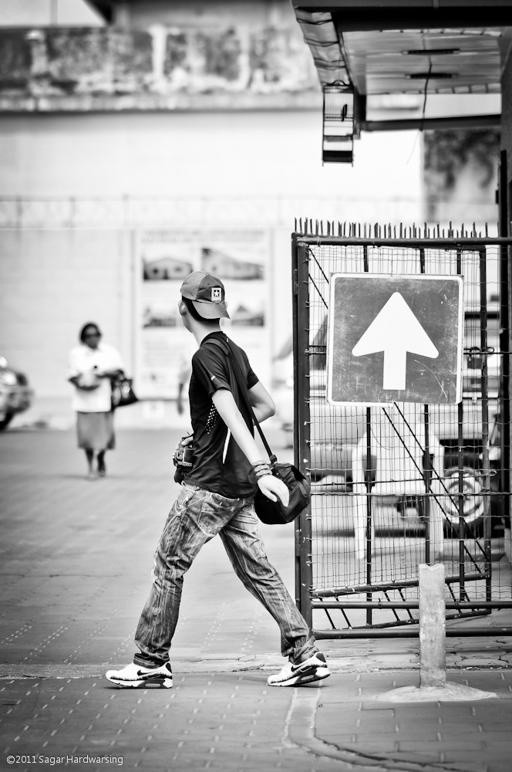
[{"x1": 250, "y1": 461, "x2": 272, "y2": 479}]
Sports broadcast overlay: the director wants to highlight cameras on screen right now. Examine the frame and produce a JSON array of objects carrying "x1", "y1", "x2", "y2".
[{"x1": 173, "y1": 437, "x2": 195, "y2": 469}]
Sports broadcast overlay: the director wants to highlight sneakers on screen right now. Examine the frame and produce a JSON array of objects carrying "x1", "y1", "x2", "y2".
[
  {"x1": 268, "y1": 652, "x2": 330, "y2": 687},
  {"x1": 105, "y1": 663, "x2": 173, "y2": 689}
]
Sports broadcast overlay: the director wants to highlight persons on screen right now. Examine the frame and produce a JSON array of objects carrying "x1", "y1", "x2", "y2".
[
  {"x1": 106, "y1": 270, "x2": 330, "y2": 689},
  {"x1": 68, "y1": 322, "x2": 128, "y2": 478}
]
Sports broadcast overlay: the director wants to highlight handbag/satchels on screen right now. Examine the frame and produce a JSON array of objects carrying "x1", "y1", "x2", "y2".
[
  {"x1": 112, "y1": 378, "x2": 138, "y2": 406},
  {"x1": 254, "y1": 462, "x2": 310, "y2": 523}
]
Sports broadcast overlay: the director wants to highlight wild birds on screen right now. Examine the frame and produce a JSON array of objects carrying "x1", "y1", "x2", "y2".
[{"x1": 342, "y1": 104, "x2": 347, "y2": 121}]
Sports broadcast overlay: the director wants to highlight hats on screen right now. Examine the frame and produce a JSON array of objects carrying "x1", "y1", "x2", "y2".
[{"x1": 180, "y1": 270, "x2": 230, "y2": 319}]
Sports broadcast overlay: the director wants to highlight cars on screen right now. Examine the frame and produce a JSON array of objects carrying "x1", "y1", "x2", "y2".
[{"x1": 0, "y1": 357, "x2": 32, "y2": 433}]
